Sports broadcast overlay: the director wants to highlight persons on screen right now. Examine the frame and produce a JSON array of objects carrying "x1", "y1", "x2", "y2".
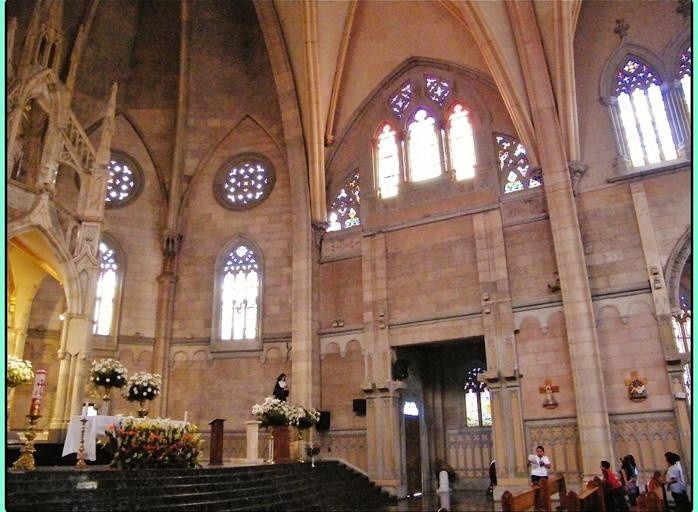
[
  {"x1": 272, "y1": 373, "x2": 289, "y2": 403},
  {"x1": 600, "y1": 454, "x2": 639, "y2": 512},
  {"x1": 527, "y1": 446, "x2": 552, "y2": 485},
  {"x1": 655, "y1": 452, "x2": 690, "y2": 512}
]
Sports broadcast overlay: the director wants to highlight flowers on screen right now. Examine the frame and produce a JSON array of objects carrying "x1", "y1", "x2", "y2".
[
  {"x1": 90, "y1": 356, "x2": 128, "y2": 388},
  {"x1": 288, "y1": 409, "x2": 320, "y2": 429},
  {"x1": 252, "y1": 395, "x2": 299, "y2": 428},
  {"x1": 7, "y1": 354, "x2": 34, "y2": 388},
  {"x1": 96, "y1": 422, "x2": 204, "y2": 471},
  {"x1": 122, "y1": 372, "x2": 161, "y2": 400}
]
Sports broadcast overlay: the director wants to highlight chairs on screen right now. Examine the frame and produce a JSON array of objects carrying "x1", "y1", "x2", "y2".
[
  {"x1": 503, "y1": 483, "x2": 541, "y2": 511},
  {"x1": 568, "y1": 481, "x2": 604, "y2": 512},
  {"x1": 539, "y1": 472, "x2": 567, "y2": 512}
]
